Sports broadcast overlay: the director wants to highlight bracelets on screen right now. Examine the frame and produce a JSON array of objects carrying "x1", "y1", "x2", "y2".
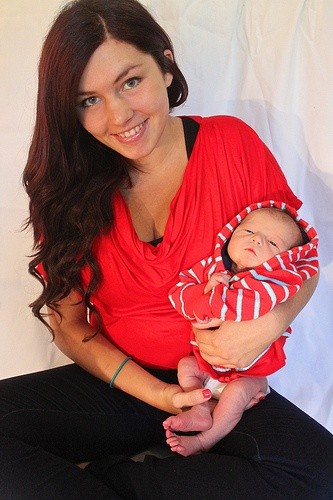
[{"x1": 109, "y1": 356, "x2": 132, "y2": 388}]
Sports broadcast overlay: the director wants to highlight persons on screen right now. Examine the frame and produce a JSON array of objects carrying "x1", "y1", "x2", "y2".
[
  {"x1": 0, "y1": 0, "x2": 333, "y2": 500},
  {"x1": 162, "y1": 200, "x2": 319, "y2": 456}
]
